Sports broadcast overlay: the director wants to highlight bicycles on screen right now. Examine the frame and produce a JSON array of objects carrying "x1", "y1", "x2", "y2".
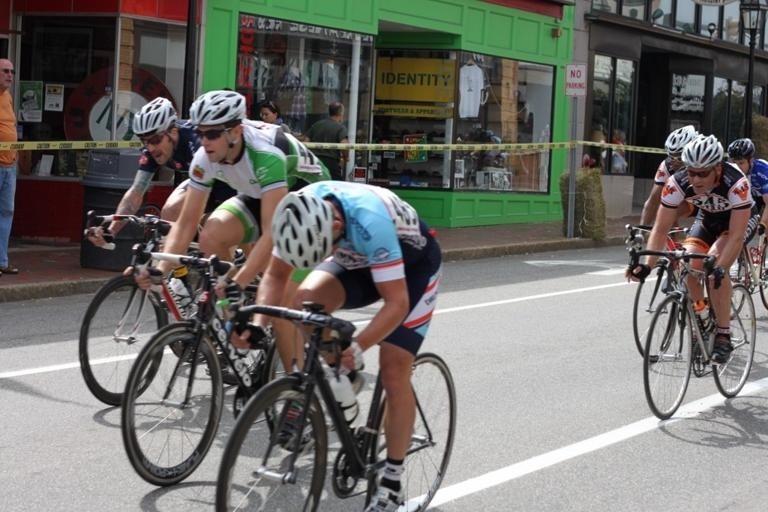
[
  {"x1": 214, "y1": 292, "x2": 457, "y2": 512},
  {"x1": 623, "y1": 205, "x2": 768, "y2": 425},
  {"x1": 77, "y1": 209, "x2": 282, "y2": 404},
  {"x1": 119, "y1": 242, "x2": 360, "y2": 487}
]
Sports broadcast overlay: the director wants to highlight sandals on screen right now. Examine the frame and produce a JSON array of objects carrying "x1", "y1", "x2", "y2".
[{"x1": 0, "y1": 265, "x2": 19, "y2": 273}]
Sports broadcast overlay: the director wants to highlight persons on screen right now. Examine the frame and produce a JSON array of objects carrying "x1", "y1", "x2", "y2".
[
  {"x1": 85, "y1": 93, "x2": 258, "y2": 354},
  {"x1": 0, "y1": 58, "x2": 21, "y2": 276},
  {"x1": 723, "y1": 137, "x2": 768, "y2": 284},
  {"x1": 626, "y1": 134, "x2": 754, "y2": 365},
  {"x1": 122, "y1": 87, "x2": 339, "y2": 445},
  {"x1": 257, "y1": 100, "x2": 293, "y2": 135},
  {"x1": 302, "y1": 101, "x2": 350, "y2": 181},
  {"x1": 631, "y1": 124, "x2": 708, "y2": 308},
  {"x1": 231, "y1": 176, "x2": 443, "y2": 512}
]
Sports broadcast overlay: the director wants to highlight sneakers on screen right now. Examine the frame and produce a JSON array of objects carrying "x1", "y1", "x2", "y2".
[
  {"x1": 365, "y1": 486, "x2": 404, "y2": 512},
  {"x1": 711, "y1": 334, "x2": 732, "y2": 362}
]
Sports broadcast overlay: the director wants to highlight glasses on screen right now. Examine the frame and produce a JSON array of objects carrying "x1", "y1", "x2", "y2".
[
  {"x1": 138, "y1": 133, "x2": 167, "y2": 144},
  {"x1": 688, "y1": 170, "x2": 715, "y2": 179},
  {"x1": 195, "y1": 127, "x2": 232, "y2": 140},
  {"x1": 1, "y1": 67, "x2": 15, "y2": 75}
]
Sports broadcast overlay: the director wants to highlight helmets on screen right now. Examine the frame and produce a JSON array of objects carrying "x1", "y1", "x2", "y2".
[
  {"x1": 187, "y1": 90, "x2": 246, "y2": 126},
  {"x1": 133, "y1": 97, "x2": 177, "y2": 137},
  {"x1": 663, "y1": 125, "x2": 756, "y2": 169},
  {"x1": 272, "y1": 189, "x2": 332, "y2": 269}
]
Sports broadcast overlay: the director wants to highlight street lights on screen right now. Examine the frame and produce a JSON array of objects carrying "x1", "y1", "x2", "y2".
[{"x1": 733, "y1": 0, "x2": 761, "y2": 145}]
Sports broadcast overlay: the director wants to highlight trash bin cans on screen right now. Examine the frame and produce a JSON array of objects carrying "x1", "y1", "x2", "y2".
[{"x1": 78, "y1": 147, "x2": 152, "y2": 272}]
